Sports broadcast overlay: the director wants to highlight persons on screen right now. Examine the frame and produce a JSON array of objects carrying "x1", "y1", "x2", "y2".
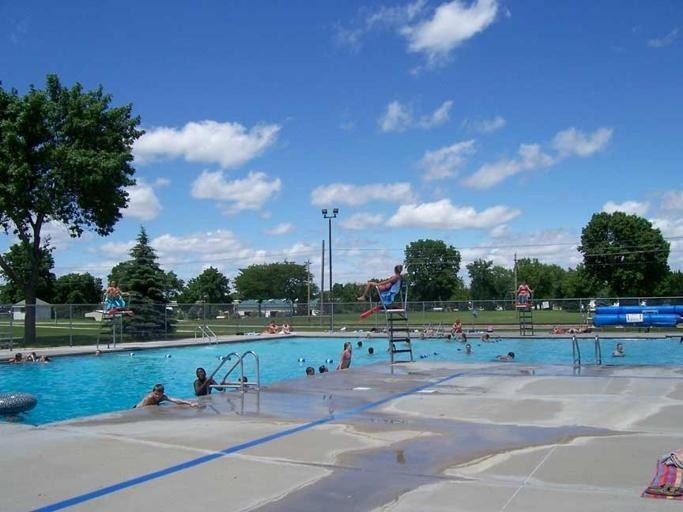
[
  {"x1": 193, "y1": 367, "x2": 248, "y2": 396},
  {"x1": 104, "y1": 280, "x2": 130, "y2": 311},
  {"x1": 612, "y1": 342, "x2": 624, "y2": 356},
  {"x1": 447, "y1": 318, "x2": 515, "y2": 362},
  {"x1": 269, "y1": 320, "x2": 289, "y2": 334},
  {"x1": 357, "y1": 264, "x2": 403, "y2": 301},
  {"x1": 9, "y1": 352, "x2": 50, "y2": 363},
  {"x1": 133, "y1": 384, "x2": 199, "y2": 407},
  {"x1": 516, "y1": 280, "x2": 533, "y2": 304},
  {"x1": 553, "y1": 326, "x2": 591, "y2": 334},
  {"x1": 306, "y1": 341, "x2": 374, "y2": 376},
  {"x1": 96, "y1": 350, "x2": 102, "y2": 356}
]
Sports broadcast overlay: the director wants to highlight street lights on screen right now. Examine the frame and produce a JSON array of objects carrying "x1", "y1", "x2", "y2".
[{"x1": 321, "y1": 206, "x2": 339, "y2": 319}]
